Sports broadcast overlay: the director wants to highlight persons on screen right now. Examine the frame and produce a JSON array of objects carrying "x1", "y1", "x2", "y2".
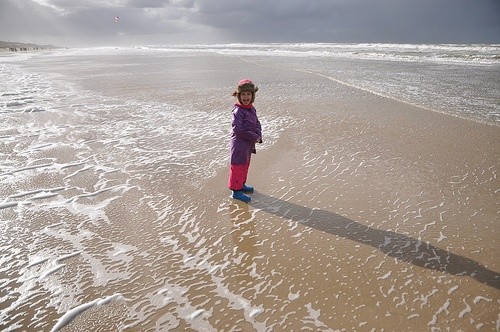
[{"x1": 228, "y1": 79, "x2": 262, "y2": 202}]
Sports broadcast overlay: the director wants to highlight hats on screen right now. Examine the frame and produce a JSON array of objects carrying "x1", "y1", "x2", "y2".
[{"x1": 238, "y1": 79, "x2": 254, "y2": 91}]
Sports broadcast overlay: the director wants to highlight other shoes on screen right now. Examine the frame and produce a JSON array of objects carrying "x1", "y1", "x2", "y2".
[
  {"x1": 233, "y1": 190, "x2": 251, "y2": 203},
  {"x1": 241, "y1": 184, "x2": 254, "y2": 192}
]
